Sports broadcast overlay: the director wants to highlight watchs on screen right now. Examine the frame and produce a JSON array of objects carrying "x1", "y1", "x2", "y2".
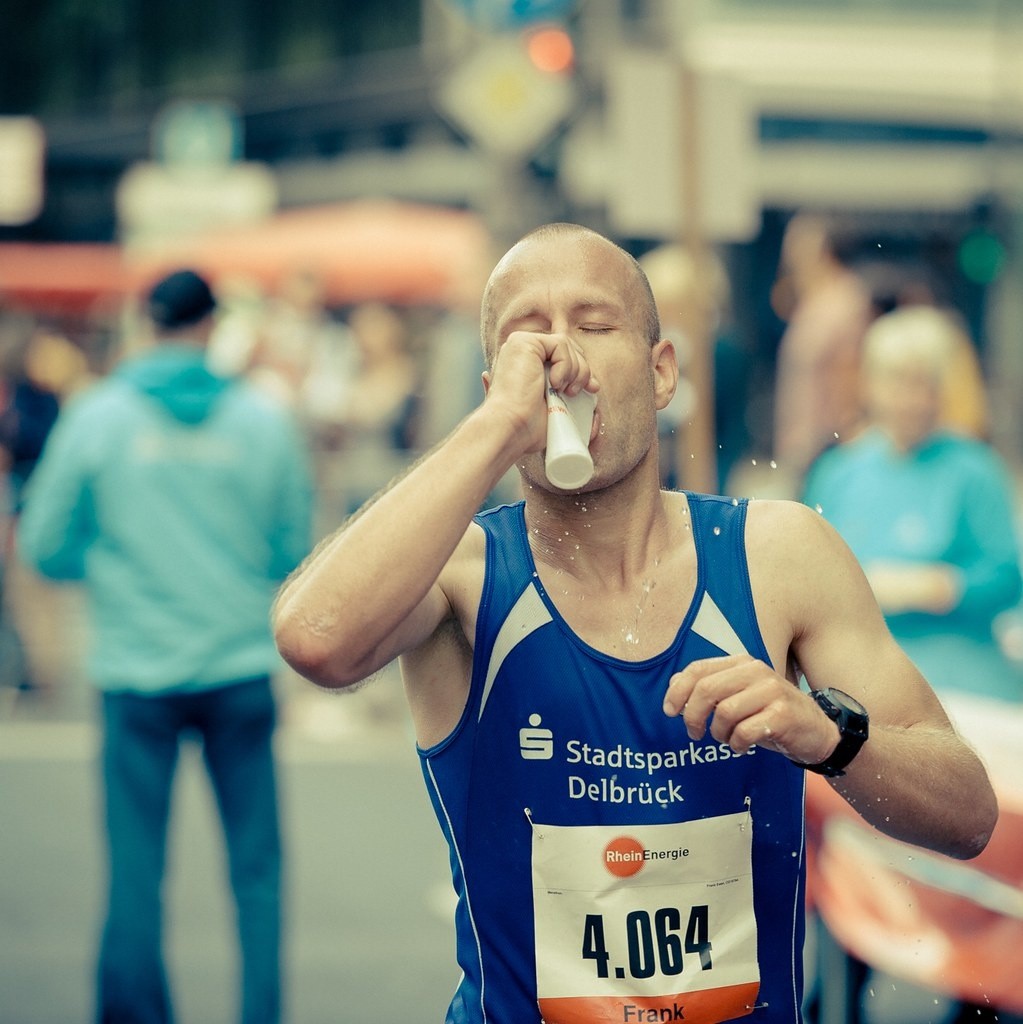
[{"x1": 783, "y1": 686, "x2": 870, "y2": 778}]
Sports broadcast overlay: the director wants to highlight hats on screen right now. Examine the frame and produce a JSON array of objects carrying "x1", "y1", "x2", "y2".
[{"x1": 151, "y1": 271, "x2": 216, "y2": 328}]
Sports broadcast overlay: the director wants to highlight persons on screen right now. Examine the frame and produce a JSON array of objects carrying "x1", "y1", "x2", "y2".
[
  {"x1": 794, "y1": 302, "x2": 1023, "y2": 707},
  {"x1": 271, "y1": 221, "x2": 999, "y2": 1024},
  {"x1": 15, "y1": 269, "x2": 312, "y2": 1023},
  {"x1": 1, "y1": 362, "x2": 61, "y2": 695}
]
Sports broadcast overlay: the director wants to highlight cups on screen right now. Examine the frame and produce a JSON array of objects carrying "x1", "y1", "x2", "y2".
[{"x1": 538, "y1": 369, "x2": 599, "y2": 491}]
